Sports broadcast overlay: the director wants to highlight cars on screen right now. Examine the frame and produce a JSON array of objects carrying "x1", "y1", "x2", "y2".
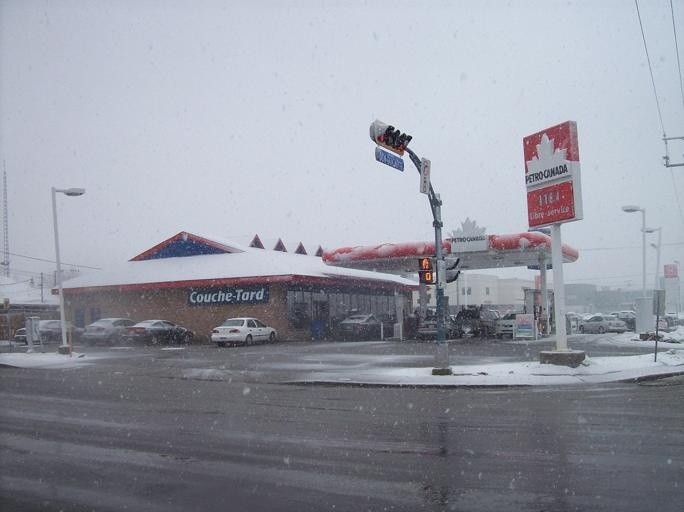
[
  {"x1": 211, "y1": 317, "x2": 278, "y2": 346},
  {"x1": 14, "y1": 317, "x2": 194, "y2": 346}
]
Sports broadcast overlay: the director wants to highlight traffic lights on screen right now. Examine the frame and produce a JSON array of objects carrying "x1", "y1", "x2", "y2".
[
  {"x1": 418, "y1": 257, "x2": 434, "y2": 283},
  {"x1": 445, "y1": 258, "x2": 460, "y2": 283},
  {"x1": 369, "y1": 119, "x2": 413, "y2": 156}
]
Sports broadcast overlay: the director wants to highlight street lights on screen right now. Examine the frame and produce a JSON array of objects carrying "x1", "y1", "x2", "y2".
[
  {"x1": 50, "y1": 187, "x2": 86, "y2": 346},
  {"x1": 621, "y1": 203, "x2": 663, "y2": 334}
]
